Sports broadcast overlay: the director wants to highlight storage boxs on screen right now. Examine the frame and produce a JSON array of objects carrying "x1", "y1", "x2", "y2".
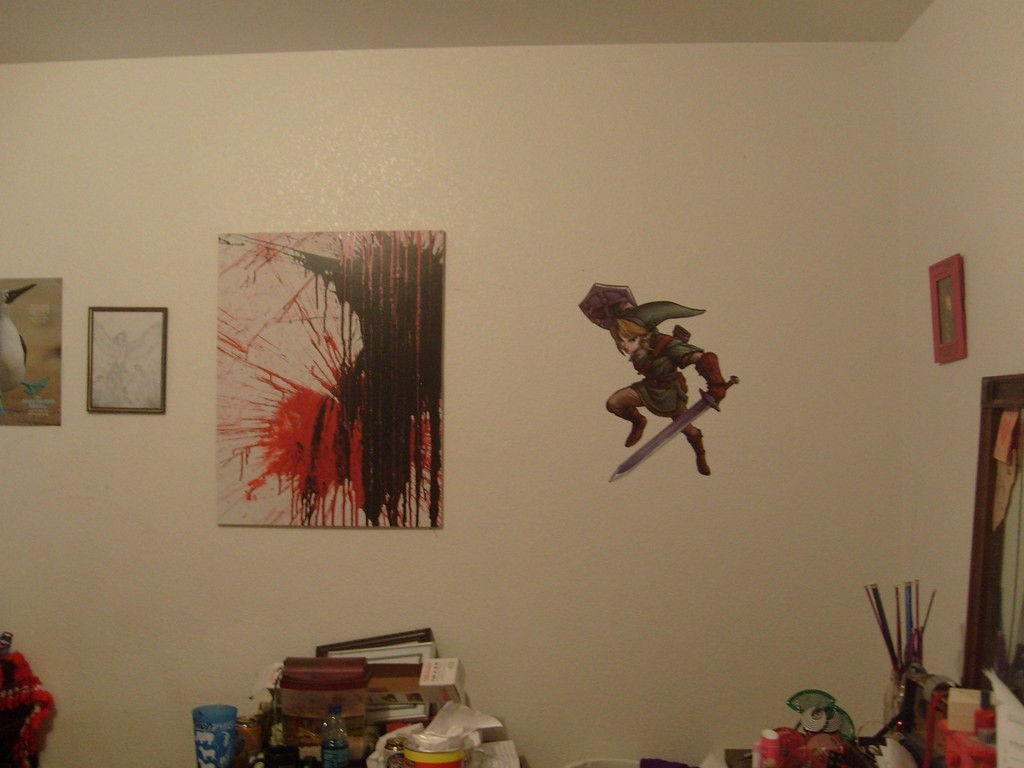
[{"x1": 276, "y1": 668, "x2": 373, "y2": 717}]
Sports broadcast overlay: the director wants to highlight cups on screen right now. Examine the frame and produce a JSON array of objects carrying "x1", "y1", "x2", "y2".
[{"x1": 192, "y1": 704, "x2": 238, "y2": 768}]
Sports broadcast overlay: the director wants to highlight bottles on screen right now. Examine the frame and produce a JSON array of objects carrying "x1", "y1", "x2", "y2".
[{"x1": 322, "y1": 703, "x2": 350, "y2": 768}]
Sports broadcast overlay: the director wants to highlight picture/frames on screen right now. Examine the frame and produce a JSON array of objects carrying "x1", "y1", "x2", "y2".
[{"x1": 85, "y1": 305, "x2": 170, "y2": 415}]
